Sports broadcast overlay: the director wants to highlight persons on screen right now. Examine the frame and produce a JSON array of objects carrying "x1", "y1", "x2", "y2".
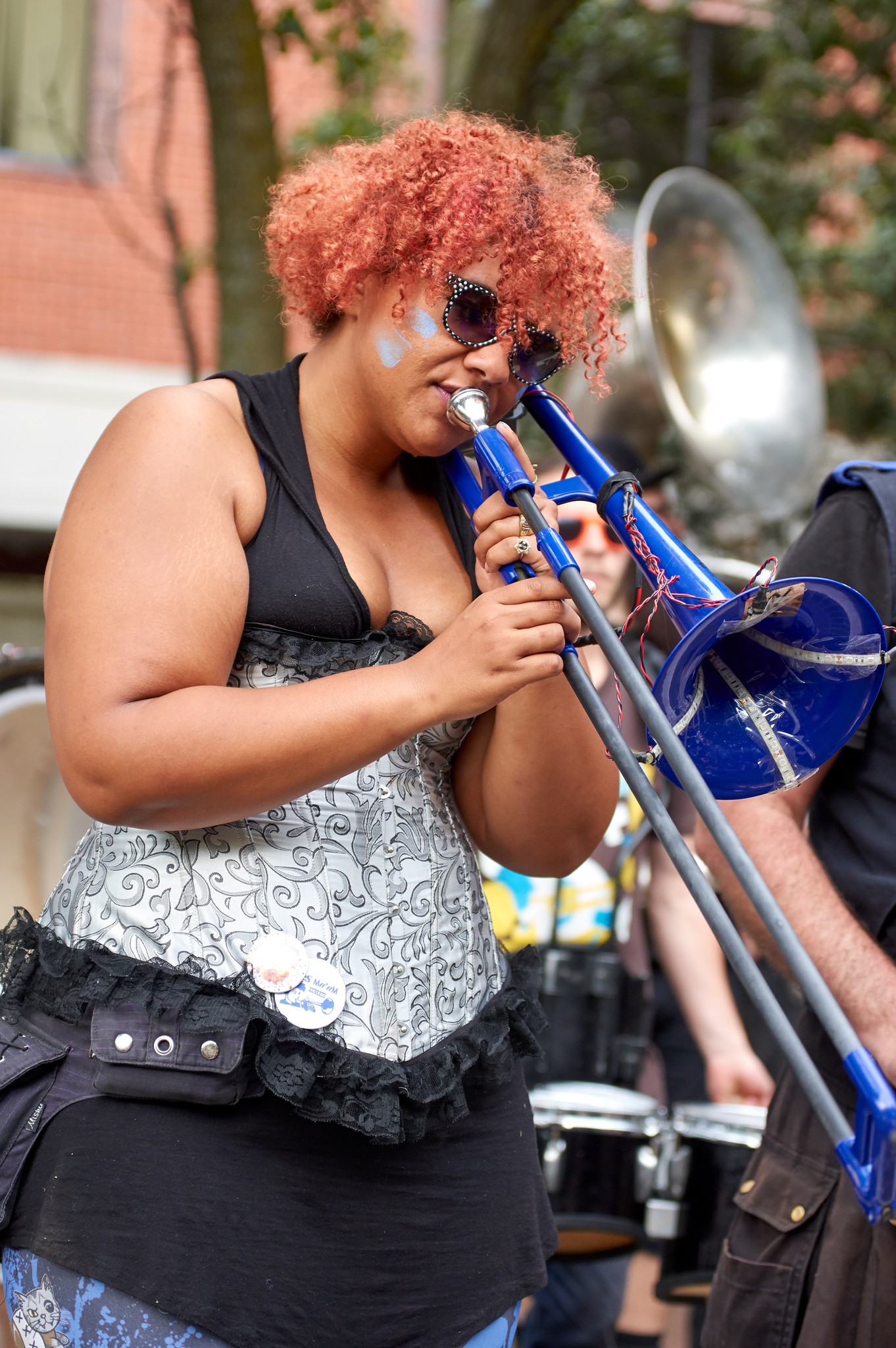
[{"x1": 0, "y1": 99, "x2": 896, "y2": 1348}]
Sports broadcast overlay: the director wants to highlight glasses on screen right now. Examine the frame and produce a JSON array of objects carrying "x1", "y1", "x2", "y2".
[
  {"x1": 419, "y1": 260, "x2": 567, "y2": 385},
  {"x1": 558, "y1": 517, "x2": 623, "y2": 545}
]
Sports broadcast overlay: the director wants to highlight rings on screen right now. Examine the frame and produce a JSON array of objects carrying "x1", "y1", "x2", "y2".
[
  {"x1": 513, "y1": 537, "x2": 529, "y2": 561},
  {"x1": 530, "y1": 464, "x2": 539, "y2": 486},
  {"x1": 517, "y1": 512, "x2": 530, "y2": 535}
]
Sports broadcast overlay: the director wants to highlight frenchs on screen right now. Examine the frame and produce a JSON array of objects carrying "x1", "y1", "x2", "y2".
[{"x1": 533, "y1": 162, "x2": 828, "y2": 589}]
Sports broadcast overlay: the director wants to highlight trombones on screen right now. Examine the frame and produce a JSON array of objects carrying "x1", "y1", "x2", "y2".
[{"x1": 430, "y1": 380, "x2": 895, "y2": 1224}]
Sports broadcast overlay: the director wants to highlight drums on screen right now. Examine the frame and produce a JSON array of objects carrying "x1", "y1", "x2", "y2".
[
  {"x1": 658, "y1": 1102, "x2": 794, "y2": 1305},
  {"x1": 520, "y1": 1085, "x2": 662, "y2": 1260}
]
